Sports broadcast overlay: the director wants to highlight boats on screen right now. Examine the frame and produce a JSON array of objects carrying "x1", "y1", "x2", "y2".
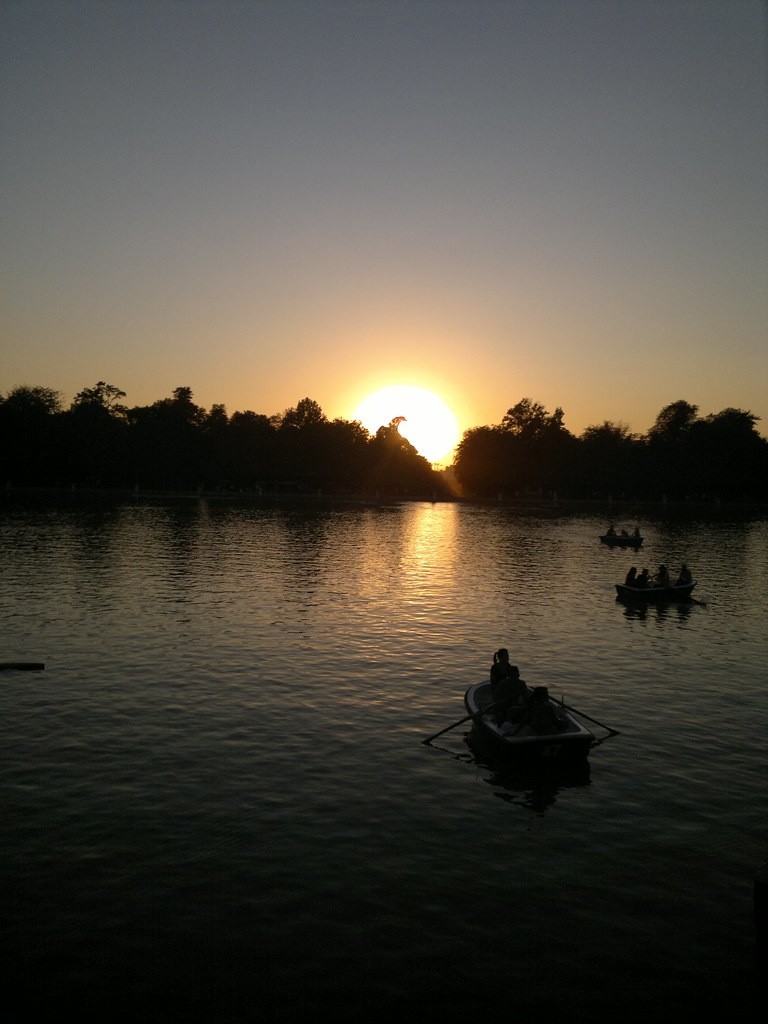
[
  {"x1": 597, "y1": 535, "x2": 644, "y2": 546},
  {"x1": 464, "y1": 678, "x2": 596, "y2": 757},
  {"x1": 613, "y1": 578, "x2": 699, "y2": 602}
]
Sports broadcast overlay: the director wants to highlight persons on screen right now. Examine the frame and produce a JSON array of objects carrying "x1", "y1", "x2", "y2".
[
  {"x1": 490, "y1": 648, "x2": 568, "y2": 736},
  {"x1": 623, "y1": 564, "x2": 693, "y2": 589},
  {"x1": 604, "y1": 524, "x2": 640, "y2": 538}
]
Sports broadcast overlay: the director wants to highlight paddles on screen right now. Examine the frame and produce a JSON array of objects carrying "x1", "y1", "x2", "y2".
[
  {"x1": 420, "y1": 701, "x2": 498, "y2": 746},
  {"x1": 529, "y1": 684, "x2": 623, "y2": 736}
]
